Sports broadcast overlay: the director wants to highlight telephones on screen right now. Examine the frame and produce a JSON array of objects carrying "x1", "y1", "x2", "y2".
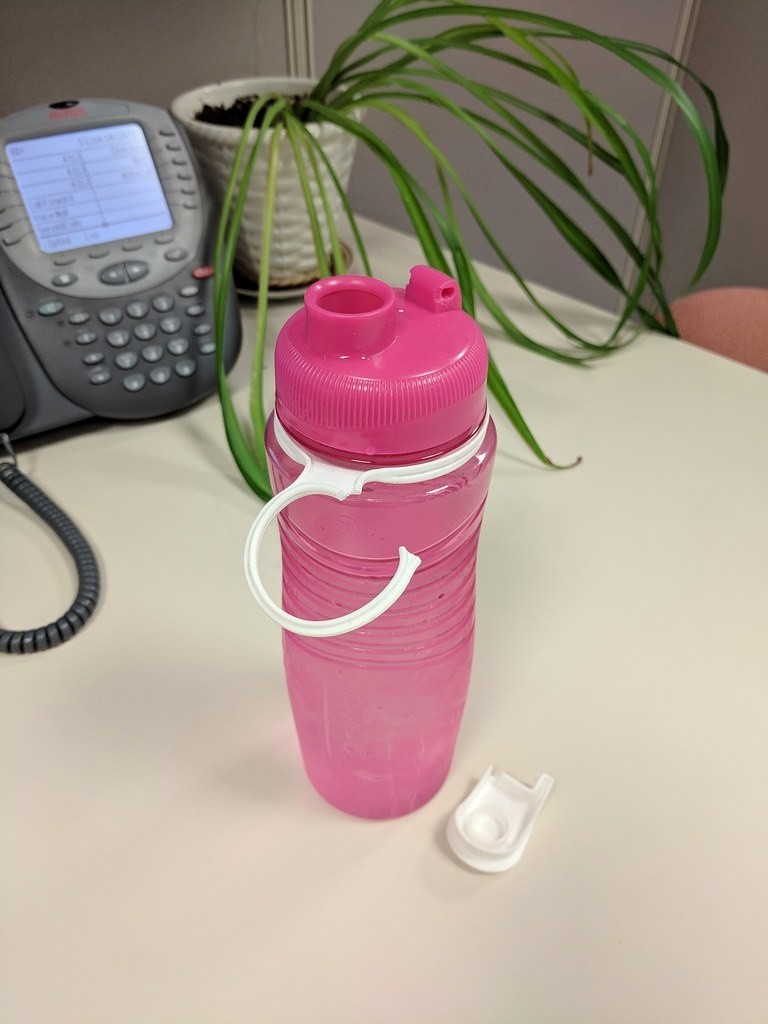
[{"x1": 0, "y1": 97, "x2": 243, "y2": 449}]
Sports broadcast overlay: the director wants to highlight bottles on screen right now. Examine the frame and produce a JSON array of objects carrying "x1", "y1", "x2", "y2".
[{"x1": 244, "y1": 261, "x2": 497, "y2": 821}]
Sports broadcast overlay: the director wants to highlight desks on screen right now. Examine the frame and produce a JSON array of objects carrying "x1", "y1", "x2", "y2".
[{"x1": 0, "y1": 208, "x2": 768, "y2": 1022}]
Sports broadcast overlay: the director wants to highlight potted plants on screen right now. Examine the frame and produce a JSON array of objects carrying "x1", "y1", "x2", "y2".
[{"x1": 170, "y1": 1, "x2": 730, "y2": 502}]
